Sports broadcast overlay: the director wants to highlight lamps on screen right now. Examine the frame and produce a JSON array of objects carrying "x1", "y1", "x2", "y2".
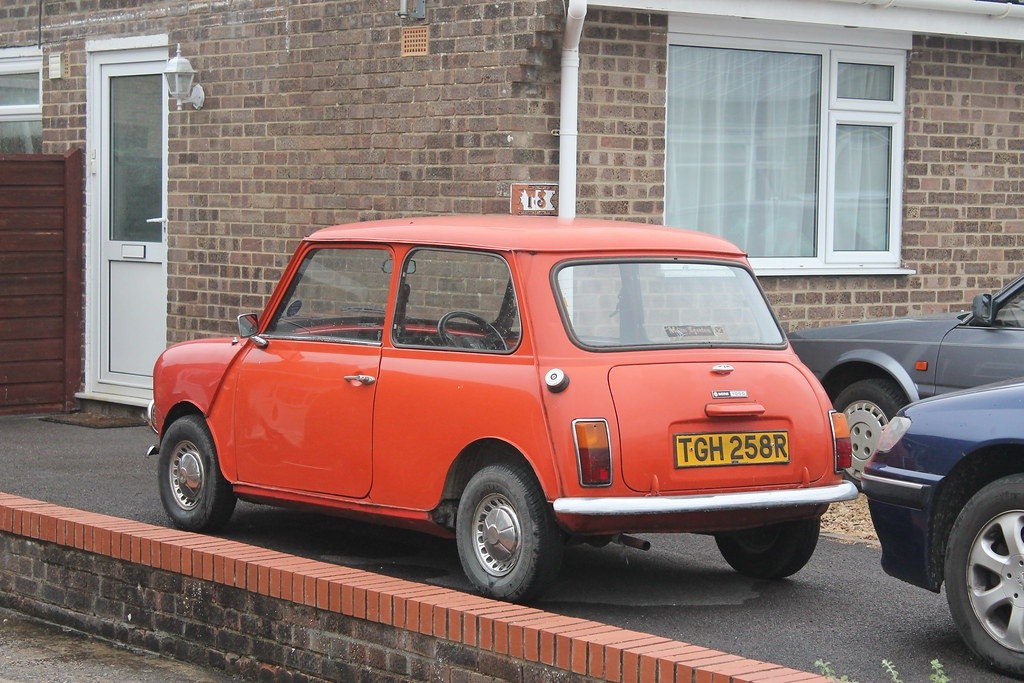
[{"x1": 162, "y1": 42, "x2": 205, "y2": 111}]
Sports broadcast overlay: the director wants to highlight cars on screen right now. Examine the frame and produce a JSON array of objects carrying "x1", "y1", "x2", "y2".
[
  {"x1": 850, "y1": 377, "x2": 1024, "y2": 680},
  {"x1": 145, "y1": 214, "x2": 858, "y2": 604},
  {"x1": 788, "y1": 271, "x2": 1024, "y2": 487}
]
